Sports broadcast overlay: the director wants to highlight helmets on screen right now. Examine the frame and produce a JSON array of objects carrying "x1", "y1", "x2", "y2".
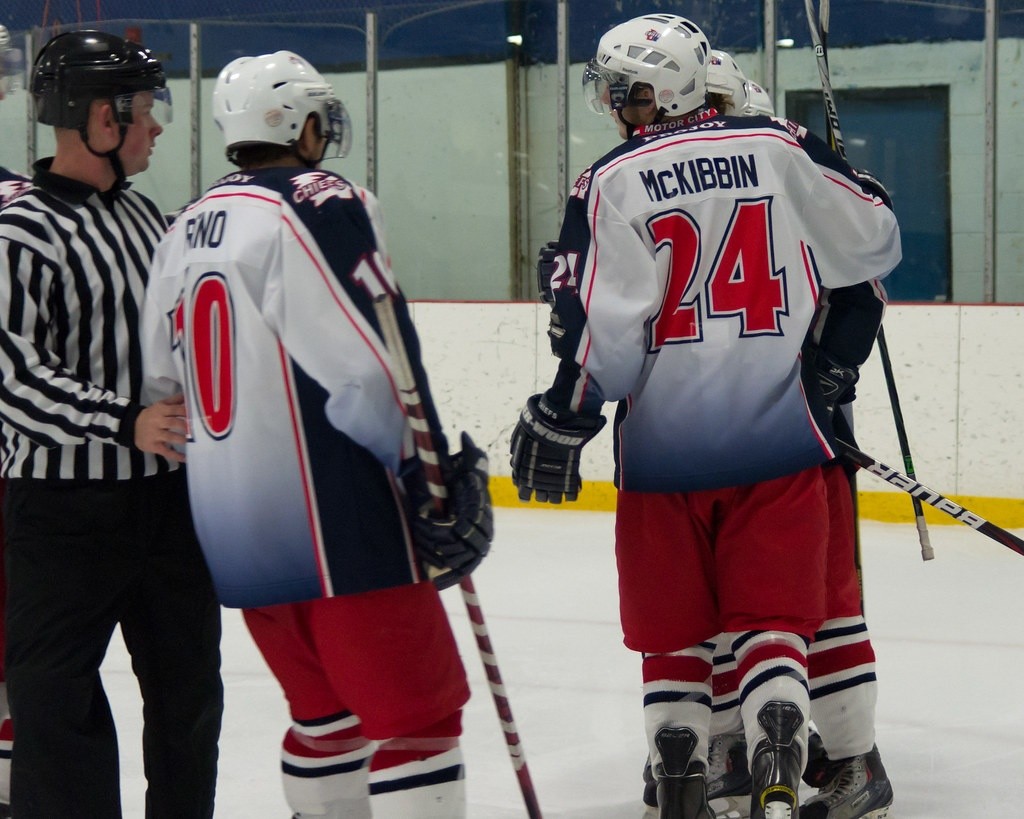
[
  {"x1": 213, "y1": 50, "x2": 336, "y2": 159},
  {"x1": 706, "y1": 49, "x2": 750, "y2": 116},
  {"x1": 742, "y1": 78, "x2": 776, "y2": 118},
  {"x1": 598, "y1": 13, "x2": 710, "y2": 117},
  {"x1": 27, "y1": 28, "x2": 173, "y2": 129}
]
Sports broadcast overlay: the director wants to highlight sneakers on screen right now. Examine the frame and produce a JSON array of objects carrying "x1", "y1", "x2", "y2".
[{"x1": 643, "y1": 730, "x2": 893, "y2": 818}]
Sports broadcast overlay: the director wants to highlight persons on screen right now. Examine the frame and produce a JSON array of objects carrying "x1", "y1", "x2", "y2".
[
  {"x1": 0, "y1": 27, "x2": 225, "y2": 818},
  {"x1": 137, "y1": 48, "x2": 492, "y2": 819},
  {"x1": 507, "y1": 12, "x2": 902, "y2": 818}
]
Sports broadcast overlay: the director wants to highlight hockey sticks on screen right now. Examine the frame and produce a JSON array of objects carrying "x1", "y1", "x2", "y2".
[
  {"x1": 799, "y1": 0, "x2": 940, "y2": 567},
  {"x1": 826, "y1": 430, "x2": 1024, "y2": 560},
  {"x1": 373, "y1": 290, "x2": 552, "y2": 818}
]
